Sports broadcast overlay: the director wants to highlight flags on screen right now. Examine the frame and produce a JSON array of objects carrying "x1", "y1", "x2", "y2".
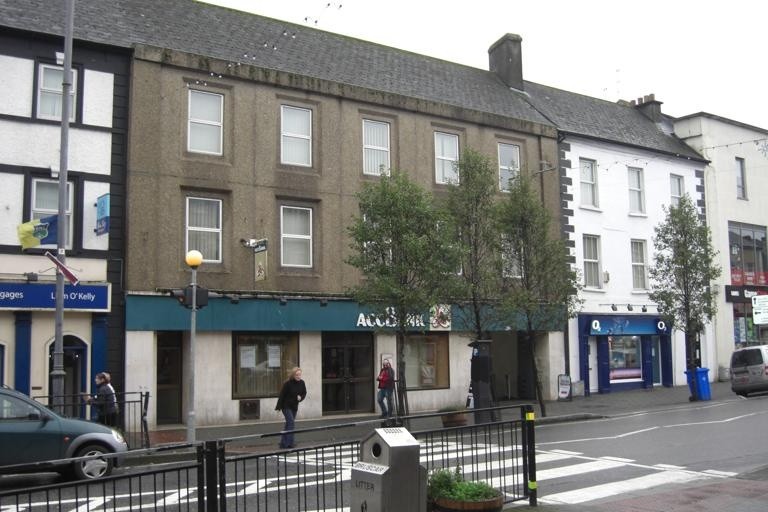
[{"x1": 17, "y1": 213, "x2": 57, "y2": 250}]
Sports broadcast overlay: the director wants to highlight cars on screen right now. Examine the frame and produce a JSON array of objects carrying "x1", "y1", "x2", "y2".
[{"x1": 1, "y1": 382, "x2": 129, "y2": 485}]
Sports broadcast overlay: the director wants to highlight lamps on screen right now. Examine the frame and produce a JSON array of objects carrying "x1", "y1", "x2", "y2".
[{"x1": 609, "y1": 303, "x2": 648, "y2": 314}]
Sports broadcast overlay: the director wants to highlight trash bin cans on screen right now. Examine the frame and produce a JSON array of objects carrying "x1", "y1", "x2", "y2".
[
  {"x1": 351, "y1": 427, "x2": 428, "y2": 512},
  {"x1": 684, "y1": 368, "x2": 711, "y2": 400}
]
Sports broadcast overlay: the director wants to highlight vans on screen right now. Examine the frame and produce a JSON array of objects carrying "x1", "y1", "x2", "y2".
[{"x1": 729, "y1": 344, "x2": 768, "y2": 398}]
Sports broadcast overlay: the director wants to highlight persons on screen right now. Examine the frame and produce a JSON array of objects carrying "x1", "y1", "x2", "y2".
[
  {"x1": 274, "y1": 366, "x2": 307, "y2": 447},
  {"x1": 79, "y1": 372, "x2": 120, "y2": 426},
  {"x1": 375, "y1": 358, "x2": 395, "y2": 417}
]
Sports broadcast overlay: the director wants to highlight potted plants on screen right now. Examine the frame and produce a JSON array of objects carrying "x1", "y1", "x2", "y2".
[
  {"x1": 428, "y1": 466, "x2": 505, "y2": 510},
  {"x1": 438, "y1": 403, "x2": 470, "y2": 428}
]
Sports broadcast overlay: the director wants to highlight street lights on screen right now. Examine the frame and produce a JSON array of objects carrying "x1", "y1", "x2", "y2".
[{"x1": 184, "y1": 248, "x2": 204, "y2": 443}]
